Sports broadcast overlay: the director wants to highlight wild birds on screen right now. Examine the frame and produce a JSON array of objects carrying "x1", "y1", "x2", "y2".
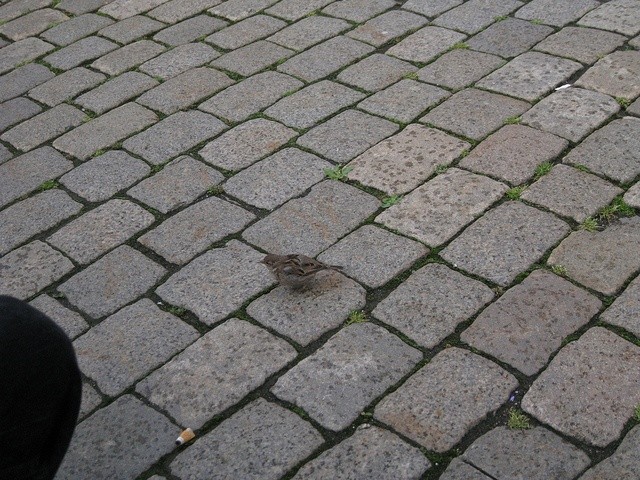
[{"x1": 256, "y1": 254, "x2": 344, "y2": 291}]
